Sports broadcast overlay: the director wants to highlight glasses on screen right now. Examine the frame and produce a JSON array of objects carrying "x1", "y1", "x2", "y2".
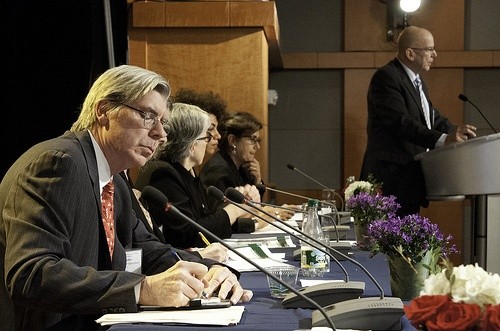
[
  {"x1": 242, "y1": 135, "x2": 262, "y2": 145},
  {"x1": 411, "y1": 47, "x2": 434, "y2": 54},
  {"x1": 119, "y1": 102, "x2": 172, "y2": 136},
  {"x1": 197, "y1": 131, "x2": 212, "y2": 142}
]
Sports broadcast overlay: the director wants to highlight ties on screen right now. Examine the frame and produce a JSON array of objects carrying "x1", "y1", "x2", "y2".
[
  {"x1": 414, "y1": 79, "x2": 429, "y2": 125},
  {"x1": 102, "y1": 181, "x2": 114, "y2": 263}
]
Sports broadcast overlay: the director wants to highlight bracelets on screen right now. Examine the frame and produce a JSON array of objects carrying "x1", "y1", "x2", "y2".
[{"x1": 275, "y1": 210, "x2": 280, "y2": 221}]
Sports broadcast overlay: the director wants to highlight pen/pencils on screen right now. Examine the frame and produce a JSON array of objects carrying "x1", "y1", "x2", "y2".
[
  {"x1": 172, "y1": 251, "x2": 208, "y2": 299},
  {"x1": 197, "y1": 231, "x2": 210, "y2": 245}
]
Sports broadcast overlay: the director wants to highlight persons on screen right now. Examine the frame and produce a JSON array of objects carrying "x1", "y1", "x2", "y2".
[
  {"x1": 127, "y1": 103, "x2": 261, "y2": 250},
  {"x1": 144, "y1": 89, "x2": 276, "y2": 235},
  {"x1": 198, "y1": 112, "x2": 302, "y2": 212},
  {"x1": 0, "y1": 65, "x2": 253, "y2": 331},
  {"x1": 354, "y1": 26, "x2": 477, "y2": 221}
]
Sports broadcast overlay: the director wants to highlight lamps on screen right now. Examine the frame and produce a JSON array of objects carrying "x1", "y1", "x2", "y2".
[{"x1": 386, "y1": 0, "x2": 422, "y2": 42}]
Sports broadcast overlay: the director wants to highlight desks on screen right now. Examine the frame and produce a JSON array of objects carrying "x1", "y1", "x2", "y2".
[{"x1": 107, "y1": 212, "x2": 417, "y2": 331}]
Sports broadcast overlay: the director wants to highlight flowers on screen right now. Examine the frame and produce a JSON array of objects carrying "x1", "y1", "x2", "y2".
[{"x1": 346, "y1": 173, "x2": 500, "y2": 331}]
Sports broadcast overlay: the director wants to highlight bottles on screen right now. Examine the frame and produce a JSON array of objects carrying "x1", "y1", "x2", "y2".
[
  {"x1": 345, "y1": 176, "x2": 356, "y2": 212},
  {"x1": 300, "y1": 199, "x2": 325, "y2": 276}
]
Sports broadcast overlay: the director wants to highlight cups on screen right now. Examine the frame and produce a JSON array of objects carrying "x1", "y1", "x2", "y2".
[
  {"x1": 264, "y1": 266, "x2": 301, "y2": 300},
  {"x1": 320, "y1": 200, "x2": 336, "y2": 227},
  {"x1": 321, "y1": 228, "x2": 330, "y2": 273}
]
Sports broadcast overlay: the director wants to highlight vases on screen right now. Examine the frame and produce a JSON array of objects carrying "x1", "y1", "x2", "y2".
[
  {"x1": 353, "y1": 217, "x2": 380, "y2": 251},
  {"x1": 388, "y1": 253, "x2": 433, "y2": 300}
]
